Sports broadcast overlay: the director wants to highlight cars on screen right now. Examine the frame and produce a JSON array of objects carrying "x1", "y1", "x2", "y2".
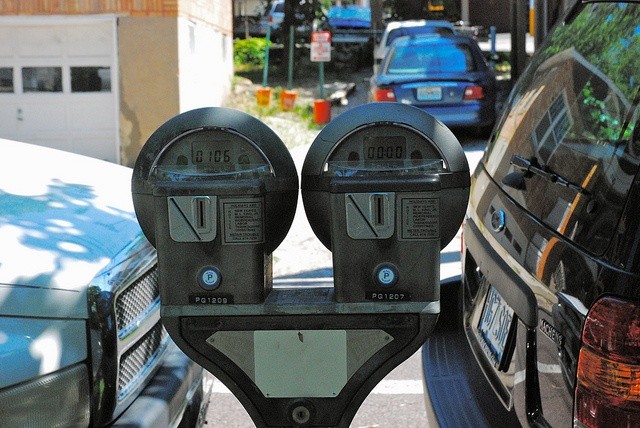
[
  {"x1": 374, "y1": 20, "x2": 456, "y2": 75},
  {"x1": 367, "y1": 33, "x2": 495, "y2": 140},
  {"x1": 318, "y1": 6, "x2": 372, "y2": 29}
]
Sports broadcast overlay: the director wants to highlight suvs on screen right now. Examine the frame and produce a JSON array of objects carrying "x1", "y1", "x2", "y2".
[
  {"x1": 465, "y1": 1, "x2": 639, "y2": 428},
  {"x1": 0, "y1": 134, "x2": 209, "y2": 428}
]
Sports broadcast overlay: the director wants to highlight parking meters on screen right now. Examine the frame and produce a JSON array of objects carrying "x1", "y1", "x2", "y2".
[{"x1": 131, "y1": 102, "x2": 471, "y2": 428}]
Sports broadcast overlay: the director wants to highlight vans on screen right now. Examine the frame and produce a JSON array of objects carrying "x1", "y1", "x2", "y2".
[{"x1": 269, "y1": 0, "x2": 311, "y2": 43}]
